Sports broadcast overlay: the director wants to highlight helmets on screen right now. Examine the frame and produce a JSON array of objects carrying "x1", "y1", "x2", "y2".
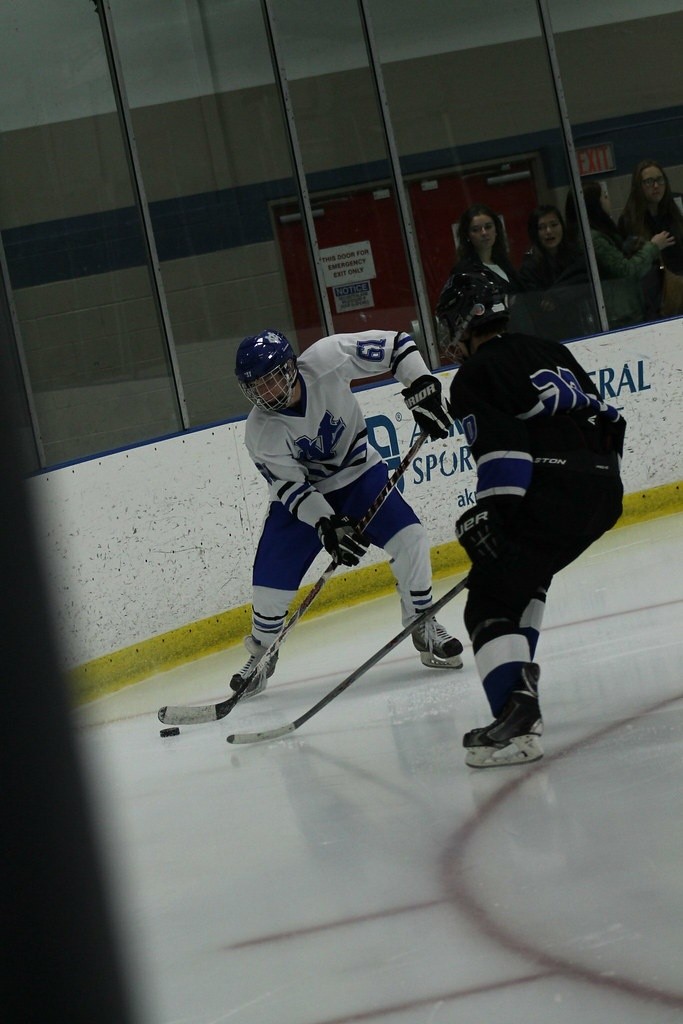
[
  {"x1": 234, "y1": 330, "x2": 298, "y2": 413},
  {"x1": 435, "y1": 274, "x2": 509, "y2": 365}
]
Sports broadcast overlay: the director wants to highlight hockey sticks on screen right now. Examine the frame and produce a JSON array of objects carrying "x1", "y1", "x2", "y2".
[
  {"x1": 224, "y1": 577, "x2": 469, "y2": 746},
  {"x1": 154, "y1": 428, "x2": 431, "y2": 727}
]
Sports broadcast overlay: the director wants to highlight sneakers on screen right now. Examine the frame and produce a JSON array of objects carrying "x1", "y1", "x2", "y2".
[
  {"x1": 464, "y1": 663, "x2": 544, "y2": 768},
  {"x1": 399, "y1": 598, "x2": 464, "y2": 668},
  {"x1": 230, "y1": 636, "x2": 278, "y2": 701}
]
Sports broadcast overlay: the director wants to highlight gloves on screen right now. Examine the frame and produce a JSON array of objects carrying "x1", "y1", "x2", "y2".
[
  {"x1": 453, "y1": 493, "x2": 523, "y2": 567},
  {"x1": 400, "y1": 376, "x2": 455, "y2": 440},
  {"x1": 314, "y1": 514, "x2": 369, "y2": 567}
]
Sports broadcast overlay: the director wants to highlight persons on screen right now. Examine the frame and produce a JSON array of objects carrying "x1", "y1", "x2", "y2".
[
  {"x1": 230, "y1": 328, "x2": 464, "y2": 698},
  {"x1": 450, "y1": 205, "x2": 537, "y2": 338},
  {"x1": 433, "y1": 271, "x2": 626, "y2": 768},
  {"x1": 565, "y1": 182, "x2": 675, "y2": 330},
  {"x1": 514, "y1": 205, "x2": 596, "y2": 339},
  {"x1": 618, "y1": 160, "x2": 683, "y2": 318}
]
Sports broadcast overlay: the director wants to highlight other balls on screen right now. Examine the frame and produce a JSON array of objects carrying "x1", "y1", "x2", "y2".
[{"x1": 159, "y1": 727, "x2": 180, "y2": 738}]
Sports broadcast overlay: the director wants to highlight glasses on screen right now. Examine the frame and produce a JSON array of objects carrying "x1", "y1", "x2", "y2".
[{"x1": 643, "y1": 176, "x2": 665, "y2": 187}]
